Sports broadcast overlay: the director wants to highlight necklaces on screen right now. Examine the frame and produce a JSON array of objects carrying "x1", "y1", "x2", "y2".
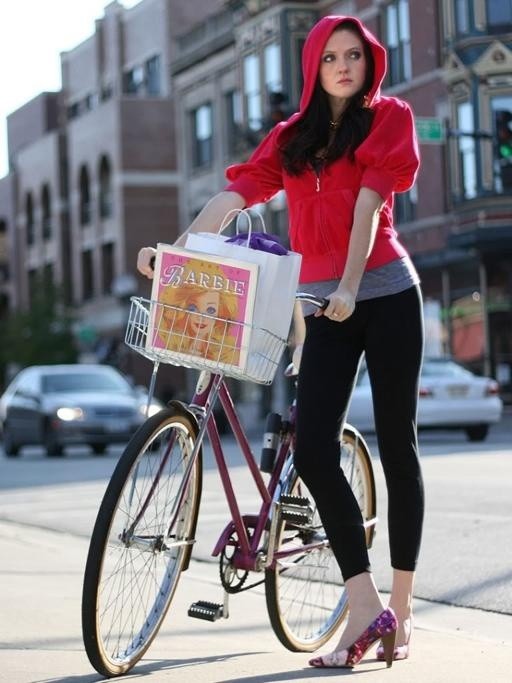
[{"x1": 327, "y1": 120, "x2": 339, "y2": 130}]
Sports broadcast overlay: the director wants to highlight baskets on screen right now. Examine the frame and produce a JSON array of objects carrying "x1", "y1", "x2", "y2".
[{"x1": 123, "y1": 297, "x2": 287, "y2": 386}]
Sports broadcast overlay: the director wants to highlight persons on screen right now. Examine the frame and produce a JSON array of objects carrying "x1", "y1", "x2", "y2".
[
  {"x1": 136, "y1": 15, "x2": 425, "y2": 668},
  {"x1": 158, "y1": 260, "x2": 238, "y2": 364}
]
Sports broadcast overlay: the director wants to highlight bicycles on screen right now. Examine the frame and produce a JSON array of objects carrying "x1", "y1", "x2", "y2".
[{"x1": 77, "y1": 254, "x2": 381, "y2": 680}]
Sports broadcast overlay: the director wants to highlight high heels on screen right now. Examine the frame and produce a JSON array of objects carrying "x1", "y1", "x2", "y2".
[
  {"x1": 310, "y1": 606, "x2": 398, "y2": 668},
  {"x1": 376, "y1": 610, "x2": 412, "y2": 661}
]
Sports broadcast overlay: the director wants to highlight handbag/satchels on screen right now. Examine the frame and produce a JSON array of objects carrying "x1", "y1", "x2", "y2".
[{"x1": 183, "y1": 207, "x2": 303, "y2": 380}]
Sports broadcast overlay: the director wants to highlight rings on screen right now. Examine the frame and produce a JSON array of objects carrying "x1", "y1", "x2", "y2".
[{"x1": 334, "y1": 312, "x2": 338, "y2": 316}]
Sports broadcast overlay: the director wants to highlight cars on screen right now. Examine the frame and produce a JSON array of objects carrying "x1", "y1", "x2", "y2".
[
  {"x1": 347, "y1": 354, "x2": 504, "y2": 444},
  {"x1": 0, "y1": 361, "x2": 168, "y2": 459}
]
